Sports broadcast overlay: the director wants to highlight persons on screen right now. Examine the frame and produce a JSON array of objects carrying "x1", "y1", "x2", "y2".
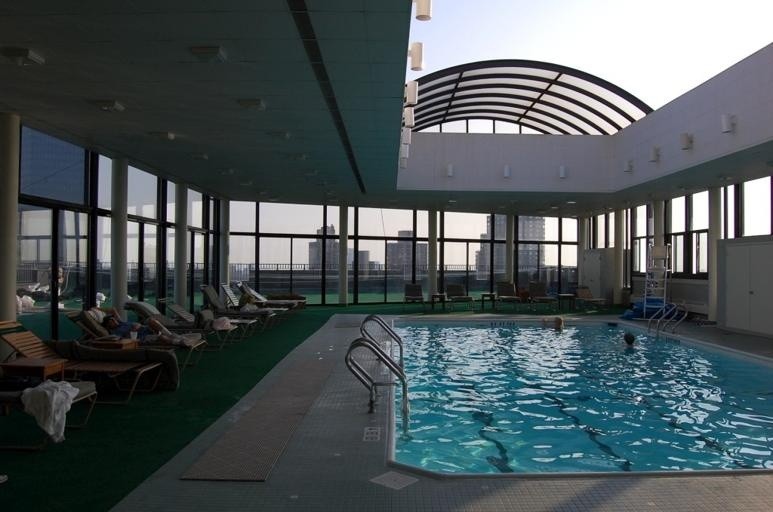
[
  {"x1": 553, "y1": 317, "x2": 562, "y2": 330},
  {"x1": 87, "y1": 306, "x2": 190, "y2": 348},
  {"x1": 623, "y1": 333, "x2": 634, "y2": 348}
]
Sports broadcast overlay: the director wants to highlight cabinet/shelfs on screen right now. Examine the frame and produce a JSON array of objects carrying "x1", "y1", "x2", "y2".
[{"x1": 716, "y1": 235, "x2": 773, "y2": 340}]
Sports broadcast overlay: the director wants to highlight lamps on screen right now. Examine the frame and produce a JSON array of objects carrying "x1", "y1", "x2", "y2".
[
  {"x1": 398, "y1": 0, "x2": 432, "y2": 170},
  {"x1": 623, "y1": 112, "x2": 738, "y2": 173},
  {"x1": 447, "y1": 161, "x2": 454, "y2": 177},
  {"x1": 559, "y1": 164, "x2": 568, "y2": 179},
  {"x1": 503, "y1": 164, "x2": 511, "y2": 180}
]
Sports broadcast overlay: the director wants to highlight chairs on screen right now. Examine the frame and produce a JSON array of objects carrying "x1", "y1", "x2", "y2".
[{"x1": 401, "y1": 279, "x2": 609, "y2": 314}]
